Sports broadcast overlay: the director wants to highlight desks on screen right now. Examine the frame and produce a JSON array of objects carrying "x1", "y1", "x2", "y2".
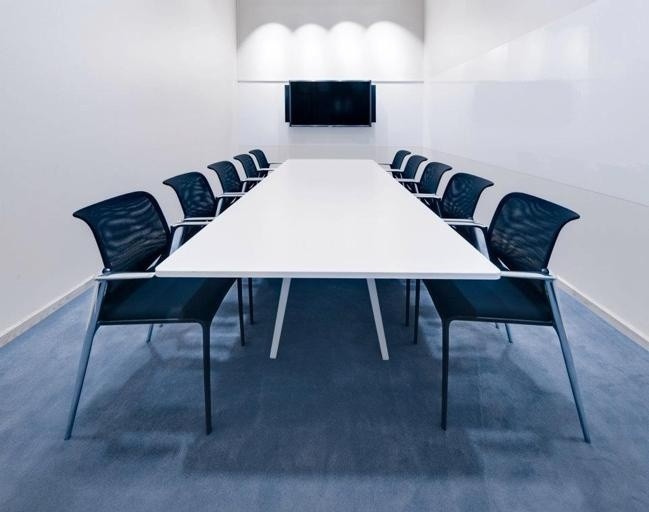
[{"x1": 155, "y1": 157, "x2": 501, "y2": 363}]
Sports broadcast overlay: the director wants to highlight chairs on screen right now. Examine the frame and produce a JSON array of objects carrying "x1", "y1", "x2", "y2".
[
  {"x1": 386, "y1": 155, "x2": 428, "y2": 192},
  {"x1": 404, "y1": 172, "x2": 501, "y2": 331},
  {"x1": 249, "y1": 150, "x2": 282, "y2": 177},
  {"x1": 377, "y1": 150, "x2": 411, "y2": 179},
  {"x1": 207, "y1": 161, "x2": 262, "y2": 208},
  {"x1": 233, "y1": 154, "x2": 275, "y2": 194},
  {"x1": 61, "y1": 190, "x2": 247, "y2": 442},
  {"x1": 398, "y1": 162, "x2": 453, "y2": 211},
  {"x1": 413, "y1": 191, "x2": 591, "y2": 442},
  {"x1": 144, "y1": 171, "x2": 254, "y2": 345}
]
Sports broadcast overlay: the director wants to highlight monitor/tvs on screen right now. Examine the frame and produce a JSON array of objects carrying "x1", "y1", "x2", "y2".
[{"x1": 285, "y1": 79, "x2": 375, "y2": 127}]
